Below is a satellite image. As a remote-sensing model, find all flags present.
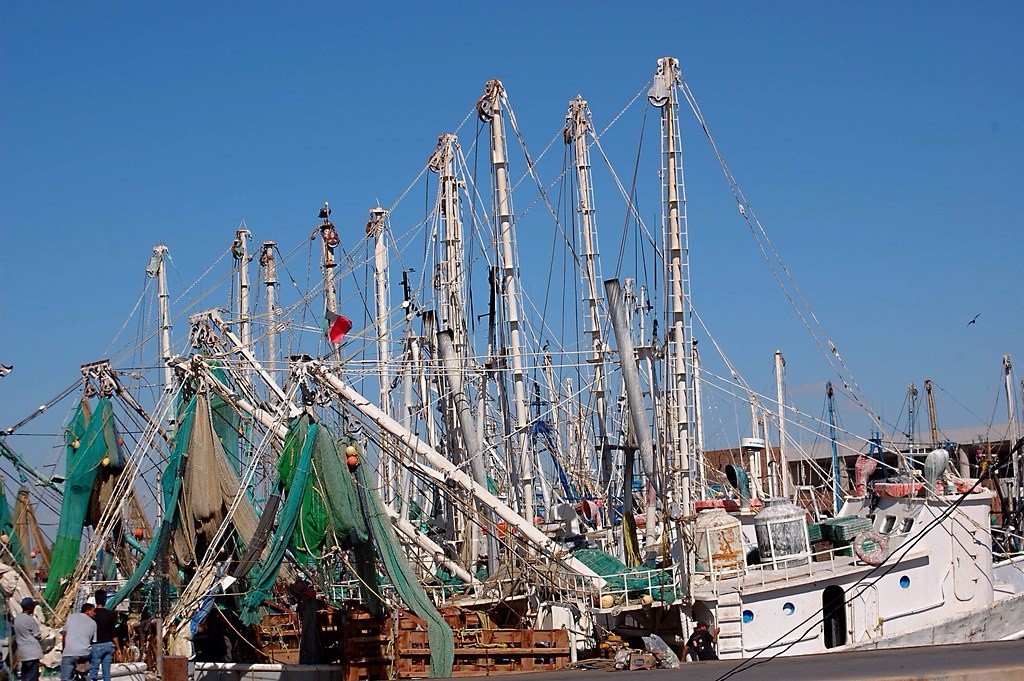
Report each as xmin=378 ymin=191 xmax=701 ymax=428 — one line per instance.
xmin=321 ymin=308 xmax=352 ymax=343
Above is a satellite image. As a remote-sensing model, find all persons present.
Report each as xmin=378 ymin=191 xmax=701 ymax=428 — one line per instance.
xmin=125 ymin=638 xmax=140 ymax=661
xmin=61 ymin=591 xmax=121 ymax=681
xmin=14 ymin=597 xmax=42 ymax=681
xmin=681 ymin=619 xmax=720 ymax=661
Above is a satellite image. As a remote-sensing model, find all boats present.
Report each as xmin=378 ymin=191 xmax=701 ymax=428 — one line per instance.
xmin=0 ymin=54 xmax=1024 ymax=660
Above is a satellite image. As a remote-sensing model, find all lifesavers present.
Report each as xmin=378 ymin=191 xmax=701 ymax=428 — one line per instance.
xmin=853 ymin=531 xmax=891 ymax=565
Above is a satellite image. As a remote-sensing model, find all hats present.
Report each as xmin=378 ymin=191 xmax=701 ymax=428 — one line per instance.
xmin=21 ymin=597 xmax=40 ymax=607
xmin=696 ymin=621 xmax=710 ymax=629
